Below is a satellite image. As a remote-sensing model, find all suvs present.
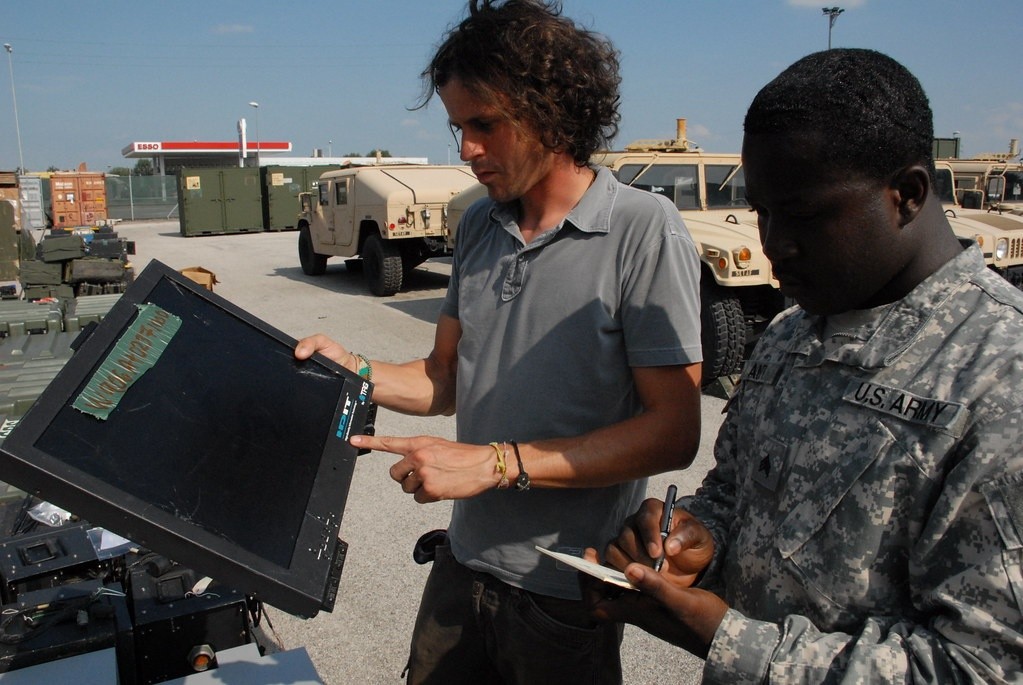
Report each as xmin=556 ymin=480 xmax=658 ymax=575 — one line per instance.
xmin=295 ymin=163 xmax=482 ymax=298
xmin=594 ymin=118 xmax=1023 ymax=393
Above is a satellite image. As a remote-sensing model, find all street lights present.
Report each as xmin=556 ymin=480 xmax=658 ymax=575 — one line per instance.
xmin=4 ymin=43 xmax=23 ymax=176
xmin=249 ymin=102 xmax=259 ymax=162
xmin=328 ymin=140 xmax=332 ymax=157
xmin=448 ymin=143 xmax=452 ymax=165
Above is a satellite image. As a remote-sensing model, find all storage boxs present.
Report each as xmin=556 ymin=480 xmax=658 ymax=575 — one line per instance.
xmin=259 ymin=164 xmax=341 ymax=233
xmin=51 ymin=174 xmax=108 ymax=228
xmin=17 ymin=176 xmax=46 ymax=230
xmin=20 ymin=224 xmax=136 ymax=300
xmin=176 ymin=266 xmax=222 ymax=292
xmin=176 ymin=168 xmax=266 ymax=237
xmin=0 ymin=172 xmax=21 ymax=232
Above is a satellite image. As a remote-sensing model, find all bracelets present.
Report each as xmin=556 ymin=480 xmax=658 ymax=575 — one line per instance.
xmin=350 ymin=350 xmax=373 ymax=381
xmin=490 ymin=438 xmax=530 ymax=492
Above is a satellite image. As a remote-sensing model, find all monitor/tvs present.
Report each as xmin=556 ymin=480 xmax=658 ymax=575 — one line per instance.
xmin=0 ymin=257 xmax=376 ymax=618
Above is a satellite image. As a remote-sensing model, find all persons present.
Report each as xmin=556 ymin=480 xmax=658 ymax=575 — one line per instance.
xmin=291 ymin=0 xmax=704 ymax=684
xmin=568 ymin=44 xmax=1023 ymax=684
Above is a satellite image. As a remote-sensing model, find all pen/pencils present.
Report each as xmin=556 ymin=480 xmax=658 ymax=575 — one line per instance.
xmin=654 ymin=483 xmax=678 ymax=572
